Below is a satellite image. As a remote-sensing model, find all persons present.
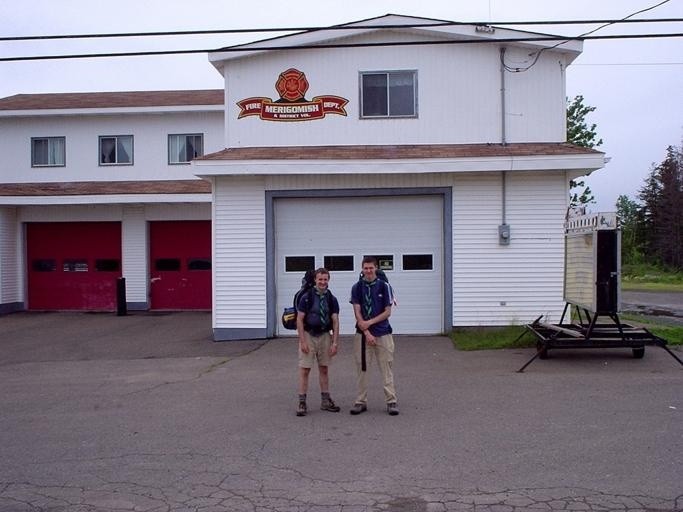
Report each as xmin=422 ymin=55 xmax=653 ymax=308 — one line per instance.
xmin=295 ymin=267 xmax=339 ymax=416
xmin=349 ymin=256 xmax=398 ymax=414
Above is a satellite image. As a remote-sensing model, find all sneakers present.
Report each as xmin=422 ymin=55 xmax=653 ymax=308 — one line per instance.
xmin=350 ymin=400 xmax=367 ymax=414
xmin=296 ymin=399 xmax=307 ymax=416
xmin=387 ymin=402 xmax=400 ymax=415
xmin=320 ymin=398 xmax=340 ymax=412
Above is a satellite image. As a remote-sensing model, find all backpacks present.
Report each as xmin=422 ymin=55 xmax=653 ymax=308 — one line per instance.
xmin=358 ymin=269 xmax=389 ymax=305
xmin=281 ymin=269 xmax=333 ymax=330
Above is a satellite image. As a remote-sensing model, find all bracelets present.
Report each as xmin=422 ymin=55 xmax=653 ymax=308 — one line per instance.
xmin=332 ymin=343 xmax=338 ymax=347
